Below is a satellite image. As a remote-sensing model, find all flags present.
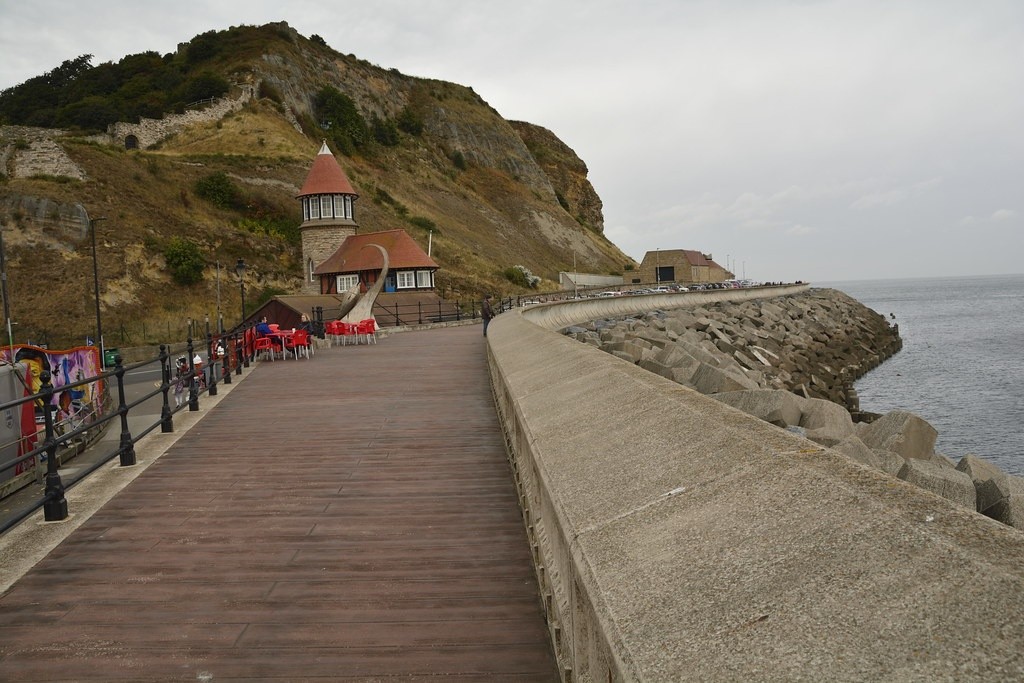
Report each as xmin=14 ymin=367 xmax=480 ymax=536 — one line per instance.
xmin=88 ymin=338 xmax=95 ymax=346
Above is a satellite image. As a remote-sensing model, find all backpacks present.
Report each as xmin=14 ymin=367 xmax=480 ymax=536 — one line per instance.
xmin=175 ymin=380 xmax=185 ymax=393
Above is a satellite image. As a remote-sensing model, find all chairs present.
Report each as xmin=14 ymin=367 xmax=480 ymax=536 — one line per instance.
xmin=324 ymin=319 xmax=377 ymax=346
xmin=252 ymin=324 xmax=314 ymax=362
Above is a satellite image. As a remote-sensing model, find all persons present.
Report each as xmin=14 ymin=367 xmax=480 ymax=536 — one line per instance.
xmin=292 ymin=313 xmax=312 ymax=355
xmin=255 ymin=316 xmax=294 ymax=355
xmin=172 ymin=373 xmax=184 ymax=408
xmin=482 ymin=294 xmax=495 ymax=337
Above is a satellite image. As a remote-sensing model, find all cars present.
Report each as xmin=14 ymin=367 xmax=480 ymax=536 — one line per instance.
xmin=600 ymin=279 xmax=759 ymax=300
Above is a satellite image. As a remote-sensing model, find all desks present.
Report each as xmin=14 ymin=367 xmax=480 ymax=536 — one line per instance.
xmin=266 ymin=333 xmax=291 ymax=362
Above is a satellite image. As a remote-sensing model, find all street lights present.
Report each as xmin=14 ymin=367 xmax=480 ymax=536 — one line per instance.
xmin=90 ymin=217 xmax=107 ymax=371
xmin=235 ymin=257 xmax=250 ymax=367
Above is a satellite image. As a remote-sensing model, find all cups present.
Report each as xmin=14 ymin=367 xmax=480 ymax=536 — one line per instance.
xmin=292 ymin=328 xmax=295 ymax=334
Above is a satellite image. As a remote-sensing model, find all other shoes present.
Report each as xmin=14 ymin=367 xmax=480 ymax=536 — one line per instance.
xmin=176 ymin=405 xmax=179 ymax=408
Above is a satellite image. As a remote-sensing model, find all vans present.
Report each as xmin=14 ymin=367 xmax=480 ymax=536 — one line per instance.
xmin=101 ymin=348 xmax=120 ymax=366
